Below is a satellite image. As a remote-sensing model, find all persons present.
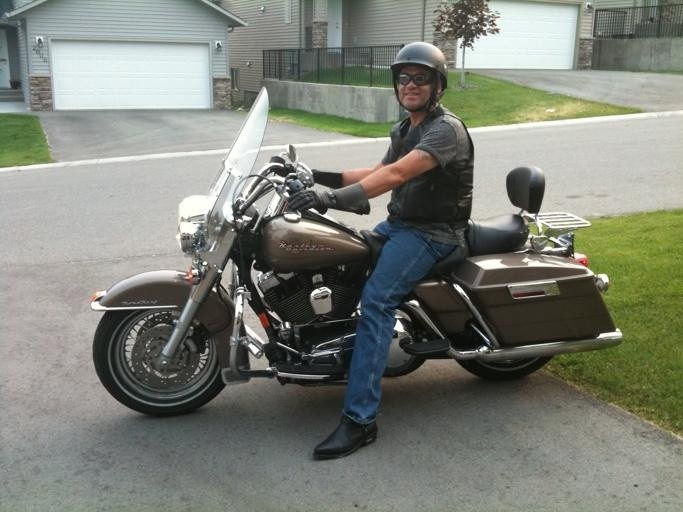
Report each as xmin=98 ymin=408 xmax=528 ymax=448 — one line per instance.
xmin=263 ymin=37 xmax=478 ymax=461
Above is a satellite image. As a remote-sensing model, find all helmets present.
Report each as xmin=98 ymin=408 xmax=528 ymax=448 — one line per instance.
xmin=390 ymin=41 xmax=448 ymax=89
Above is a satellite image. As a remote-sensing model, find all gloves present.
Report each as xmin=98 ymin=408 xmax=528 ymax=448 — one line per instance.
xmin=286 ymin=183 xmax=371 ymax=218
xmin=310 ymin=168 xmax=342 ymax=189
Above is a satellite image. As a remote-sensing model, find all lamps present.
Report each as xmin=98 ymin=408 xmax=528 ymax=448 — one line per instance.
xmin=36 ymin=36 xmax=43 ymax=47
xmin=215 ymin=41 xmax=222 ymax=52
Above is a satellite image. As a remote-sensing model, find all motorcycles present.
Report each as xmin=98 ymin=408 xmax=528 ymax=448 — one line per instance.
xmin=86 ymin=86 xmax=623 ymax=419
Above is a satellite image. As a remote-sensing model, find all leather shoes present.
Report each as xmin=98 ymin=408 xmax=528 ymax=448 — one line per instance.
xmin=312 ymin=417 xmax=379 ymax=460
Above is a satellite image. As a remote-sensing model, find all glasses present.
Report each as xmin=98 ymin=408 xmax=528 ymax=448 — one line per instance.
xmin=396 ymin=72 xmax=435 ymax=86
